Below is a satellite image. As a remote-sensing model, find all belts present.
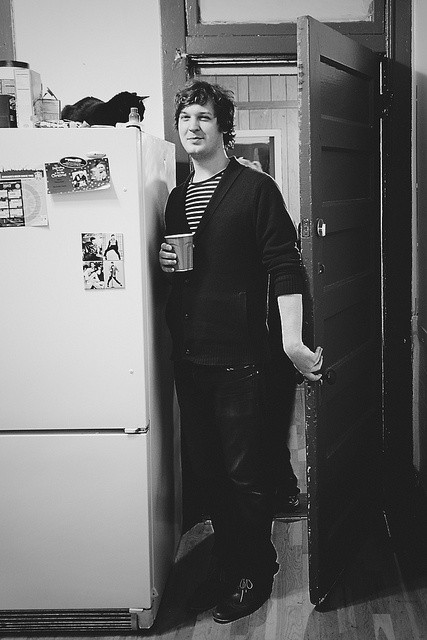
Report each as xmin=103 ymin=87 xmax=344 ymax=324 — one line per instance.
xmin=178 ymin=362 xmax=256 ymax=384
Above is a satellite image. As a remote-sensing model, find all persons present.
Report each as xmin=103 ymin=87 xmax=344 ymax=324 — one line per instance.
xmin=158 ymin=83 xmax=325 ymax=624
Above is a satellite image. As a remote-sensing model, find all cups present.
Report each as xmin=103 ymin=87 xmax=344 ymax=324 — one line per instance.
xmin=164 ymin=228 xmax=196 ymax=275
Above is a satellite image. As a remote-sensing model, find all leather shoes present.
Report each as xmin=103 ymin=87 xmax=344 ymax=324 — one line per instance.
xmin=189 ymin=571 xmax=227 ymax=614
xmin=213 ymin=574 xmax=272 ymax=625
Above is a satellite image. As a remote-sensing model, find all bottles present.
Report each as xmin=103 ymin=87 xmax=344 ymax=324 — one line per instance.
xmin=129 ymin=106 xmax=140 ymax=127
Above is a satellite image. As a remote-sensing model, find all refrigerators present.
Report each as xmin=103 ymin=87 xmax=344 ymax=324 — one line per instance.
xmin=1 ymin=126 xmax=187 ymax=635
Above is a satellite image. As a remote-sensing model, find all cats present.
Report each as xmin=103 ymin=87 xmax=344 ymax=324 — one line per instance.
xmin=60 ymin=90 xmax=150 ymax=128
